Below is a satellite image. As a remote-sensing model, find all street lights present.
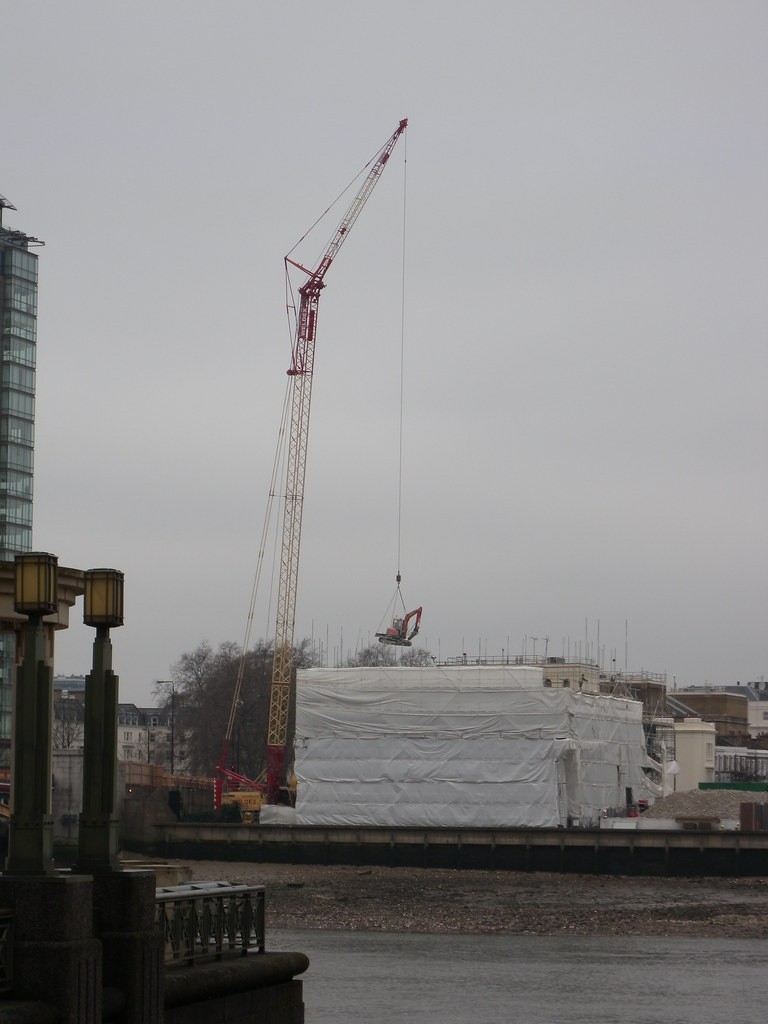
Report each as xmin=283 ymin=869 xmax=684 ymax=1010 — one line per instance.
xmin=4 ymin=550 xmax=61 ymax=876
xmin=73 ymin=565 xmax=127 ymax=874
xmin=62 ymin=698 xmax=79 ymax=748
xmin=142 ymin=726 xmax=150 ymax=765
xmin=156 ymin=680 xmax=175 ymax=776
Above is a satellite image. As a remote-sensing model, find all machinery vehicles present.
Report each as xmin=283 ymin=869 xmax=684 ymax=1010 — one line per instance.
xmin=205 ymin=111 xmax=413 ymax=826
xmin=375 ymin=605 xmax=424 ymax=647
